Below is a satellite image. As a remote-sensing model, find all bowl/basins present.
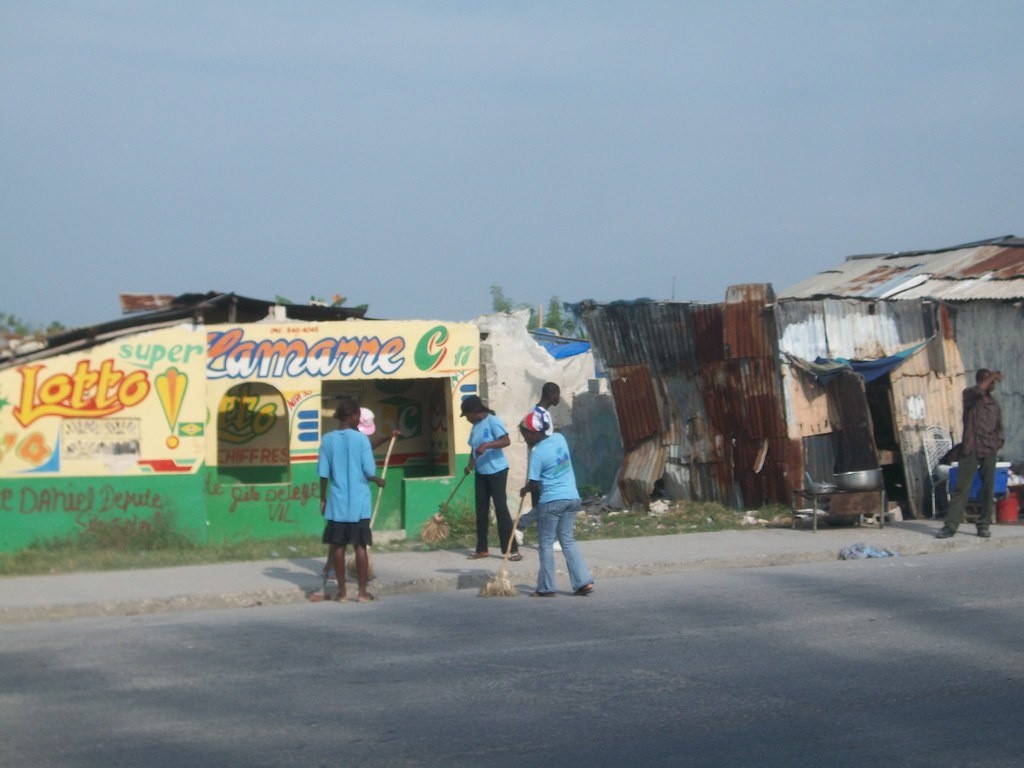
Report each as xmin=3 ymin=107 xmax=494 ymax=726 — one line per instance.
xmin=833 ymin=468 xmax=882 ymax=491
xmin=810 ymin=484 xmax=837 ymax=494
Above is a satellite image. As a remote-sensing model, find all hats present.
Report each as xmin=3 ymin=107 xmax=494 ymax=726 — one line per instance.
xmin=460 ymin=396 xmax=481 ymax=417
xmin=357 ymin=407 xmax=376 ymax=435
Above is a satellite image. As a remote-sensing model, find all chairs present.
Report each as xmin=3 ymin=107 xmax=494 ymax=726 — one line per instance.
xmin=920 ymin=426 xmax=998 ymax=524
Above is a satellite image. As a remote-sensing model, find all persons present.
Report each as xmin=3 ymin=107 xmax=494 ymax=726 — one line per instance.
xmin=935 ymin=368 xmax=1006 ymax=538
xmin=519 ymin=405 xmax=596 ymax=596
xmin=459 ymin=395 xmax=523 ymax=561
xmin=513 ymin=381 xmax=562 ymax=552
xmin=316 ymin=401 xmax=386 ymax=602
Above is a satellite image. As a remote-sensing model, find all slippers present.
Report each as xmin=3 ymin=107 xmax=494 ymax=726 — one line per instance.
xmin=530 ymin=591 xmax=556 ymax=597
xmin=574 ymin=588 xmax=593 ymax=595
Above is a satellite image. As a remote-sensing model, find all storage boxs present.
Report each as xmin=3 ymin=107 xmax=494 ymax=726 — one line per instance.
xmin=947 ymin=461 xmax=1012 ymax=503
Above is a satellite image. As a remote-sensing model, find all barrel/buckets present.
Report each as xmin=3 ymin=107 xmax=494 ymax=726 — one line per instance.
xmin=996 ymin=492 xmax=1019 ymax=523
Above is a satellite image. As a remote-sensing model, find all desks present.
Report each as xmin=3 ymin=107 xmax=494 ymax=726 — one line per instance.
xmin=790 ymin=488 xmax=885 ymax=534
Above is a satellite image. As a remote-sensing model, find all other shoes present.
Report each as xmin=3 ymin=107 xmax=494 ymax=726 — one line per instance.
xmin=978 ymin=530 xmax=991 ymax=537
xmin=937 ymin=526 xmax=953 ymax=538
xmin=337 ymin=592 xmax=347 ymax=602
xmin=361 ymin=593 xmax=374 ymax=602
xmin=509 ymin=552 xmax=521 ymax=561
xmin=467 ymin=552 xmax=488 ymax=559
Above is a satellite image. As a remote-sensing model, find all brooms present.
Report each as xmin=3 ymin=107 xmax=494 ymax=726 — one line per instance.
xmin=420 ymin=454 xmax=480 ymax=544
xmin=477 ymin=479 xmax=530 ymax=598
xmin=309 ymin=543 xmax=334 ymax=603
xmin=345 ymin=428 xmax=402 ymax=582
